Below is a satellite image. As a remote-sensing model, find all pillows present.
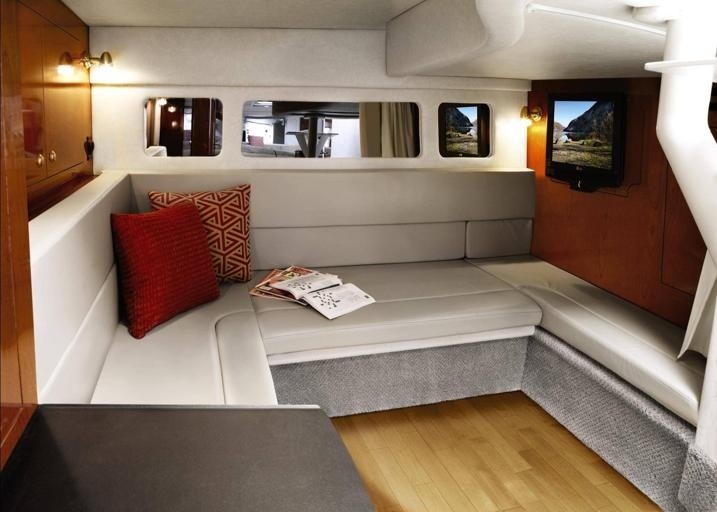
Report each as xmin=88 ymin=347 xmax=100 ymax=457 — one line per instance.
xmin=110 ymin=201 xmax=220 ymax=337
xmin=148 ymin=185 xmax=251 ymax=284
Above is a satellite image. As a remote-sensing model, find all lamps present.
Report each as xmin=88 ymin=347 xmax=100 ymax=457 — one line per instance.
xmin=56 ymin=50 xmax=82 ymax=78
xmin=520 ymin=101 xmax=541 ymax=124
xmin=82 ymin=50 xmax=114 ymax=69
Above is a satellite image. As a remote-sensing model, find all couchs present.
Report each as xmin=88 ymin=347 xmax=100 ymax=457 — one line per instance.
xmin=28 ymin=163 xmax=717 ymax=509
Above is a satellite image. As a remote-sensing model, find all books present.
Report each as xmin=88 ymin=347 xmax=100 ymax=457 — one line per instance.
xmin=249 ymin=264 xmax=376 ymax=321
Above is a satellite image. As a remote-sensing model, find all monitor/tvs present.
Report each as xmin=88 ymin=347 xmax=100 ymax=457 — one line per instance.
xmin=543 ymin=92 xmax=628 ymax=192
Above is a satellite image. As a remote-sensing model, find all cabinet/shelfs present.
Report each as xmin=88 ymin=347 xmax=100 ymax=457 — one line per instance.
xmin=15 ymin=1 xmax=89 ymax=196
xmin=0 ymin=0 xmax=37 ymax=484
xmin=661 ymin=148 xmax=707 ymax=296
